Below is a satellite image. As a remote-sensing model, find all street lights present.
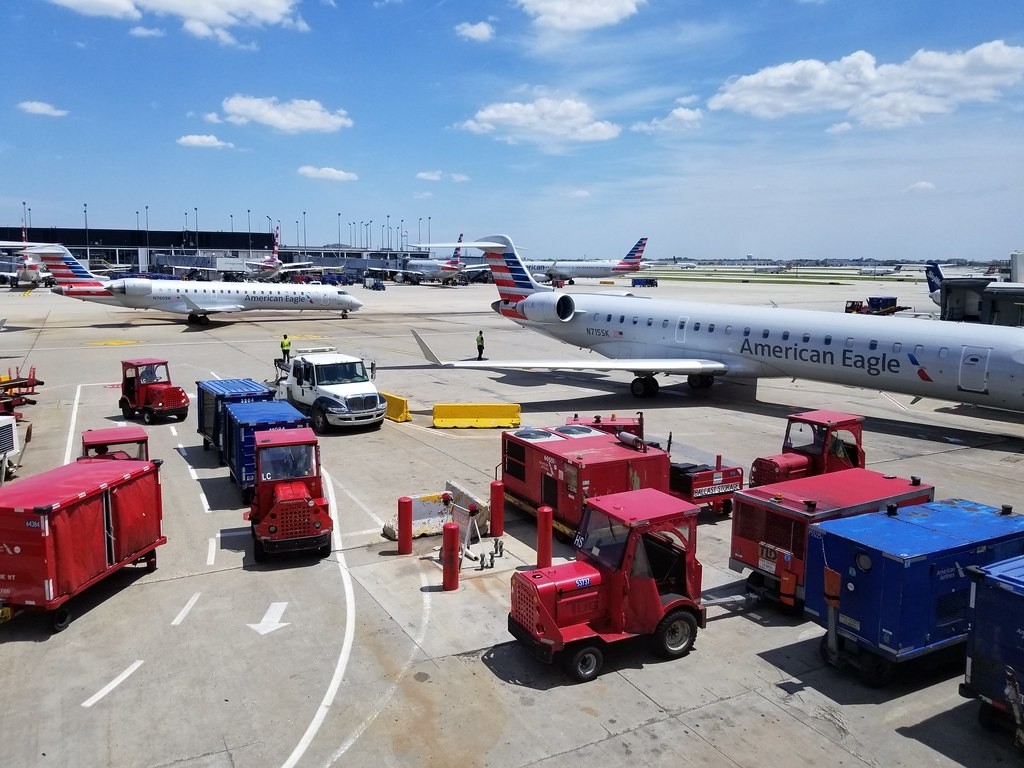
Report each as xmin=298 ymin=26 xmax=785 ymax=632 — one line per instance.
xmin=22 ymin=202 xmax=32 ymax=242
xmin=83 ymin=203 xmax=89 ymax=260
xmin=184 ymin=212 xmax=188 ymax=240
xmin=195 ymin=208 xmax=198 ymax=249
xmin=135 ymin=211 xmax=139 ymax=231
xmin=230 ymin=214 xmax=233 ymax=232
xmin=266 ymin=215 xmax=272 ymax=233
xmin=247 ymin=209 xmax=251 ymax=249
xmin=337 ymin=213 xmax=341 ymax=251
xmin=348 ymin=214 xmax=431 ymax=249
xmin=145 ymin=206 xmax=148 ymax=248
xmin=295 ymin=211 xmax=306 ymax=256
xmin=277 ymin=219 xmax=281 ymax=246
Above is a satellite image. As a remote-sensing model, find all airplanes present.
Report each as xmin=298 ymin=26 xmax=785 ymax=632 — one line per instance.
xmin=244 ymin=257 xmax=318 ymax=284
xmin=17 ymin=244 xmax=365 ymax=326
xmin=522 ymin=238 xmax=660 ymax=285
xmin=0 ymin=257 xmax=53 ymax=288
xmin=753 ymin=262 xmax=793 ymax=274
xmin=370 ymin=234 xmax=492 ymax=286
xmin=857 ymin=264 xmax=901 ymax=276
xmin=403 ymin=235 xmax=1024 ymax=412
xmin=666 ymin=256 xmax=696 ymax=270
xmin=892 ymin=261 xmax=1024 ymax=307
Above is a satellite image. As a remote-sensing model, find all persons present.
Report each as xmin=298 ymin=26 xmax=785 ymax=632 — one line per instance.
xmin=94 ymin=446 xmax=117 ymax=460
xmin=476 ymin=330 xmax=485 ymax=360
xmin=281 ymin=334 xmax=291 ymax=364
xmin=141 ymin=367 xmax=155 ymax=383
xmin=282 ymin=445 xmax=310 ymax=476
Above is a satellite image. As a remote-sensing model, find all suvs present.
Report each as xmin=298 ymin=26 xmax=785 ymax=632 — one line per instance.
xmin=287 ymin=353 xmax=388 ymax=433
